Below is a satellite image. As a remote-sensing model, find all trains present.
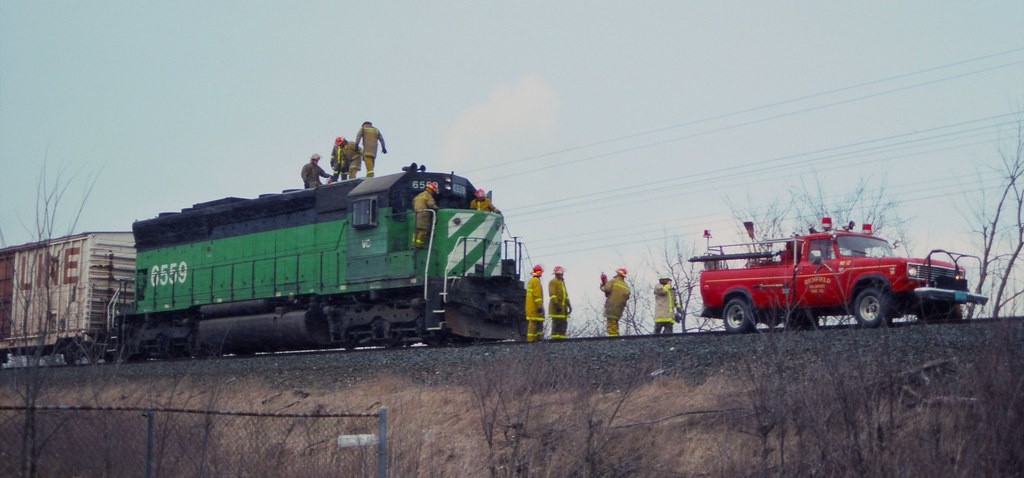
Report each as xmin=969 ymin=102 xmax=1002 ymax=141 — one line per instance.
xmin=0 ymin=162 xmax=526 ymax=364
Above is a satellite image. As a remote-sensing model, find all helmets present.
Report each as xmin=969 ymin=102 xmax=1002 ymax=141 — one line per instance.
xmin=310 ymin=154 xmax=320 ymax=160
xmin=531 ymin=264 xmax=544 ymax=273
xmin=552 ymin=266 xmax=564 ymax=273
xmin=428 ymin=183 xmax=436 ymax=190
xmin=616 ymin=269 xmax=628 ymax=277
xmin=335 ymin=137 xmax=345 ymax=145
xmin=475 ymin=189 xmax=485 ymax=197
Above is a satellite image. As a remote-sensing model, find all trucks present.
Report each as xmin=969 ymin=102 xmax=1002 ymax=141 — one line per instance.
xmin=688 ymin=217 xmax=989 ymax=327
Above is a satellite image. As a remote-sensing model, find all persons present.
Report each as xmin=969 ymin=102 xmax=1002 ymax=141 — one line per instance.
xmin=336 ymin=137 xmax=364 ymax=180
xmin=469 ymin=188 xmax=503 ymax=215
xmin=525 ymin=264 xmax=546 ymax=342
xmin=301 ymin=153 xmax=333 ymax=190
xmin=330 ymin=145 xmax=348 ymax=183
xmin=599 ymin=268 xmax=630 ymax=337
xmin=652 ymin=271 xmax=685 ymax=334
xmin=548 ymin=265 xmax=572 ymax=339
xmin=411 ymin=182 xmax=437 ymax=249
xmin=356 ymin=121 xmax=388 ymax=178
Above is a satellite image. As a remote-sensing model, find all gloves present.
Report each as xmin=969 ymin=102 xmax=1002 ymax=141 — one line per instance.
xmin=382 ymin=149 xmax=387 ymax=154
xmin=356 ymin=145 xmax=358 ymax=151
xmin=677 ymin=308 xmax=687 ymax=316
xmin=675 ymin=314 xmax=682 ymax=323
xmin=538 ymin=308 xmax=543 ymax=313
xmin=555 ymin=304 xmax=561 ymax=312
